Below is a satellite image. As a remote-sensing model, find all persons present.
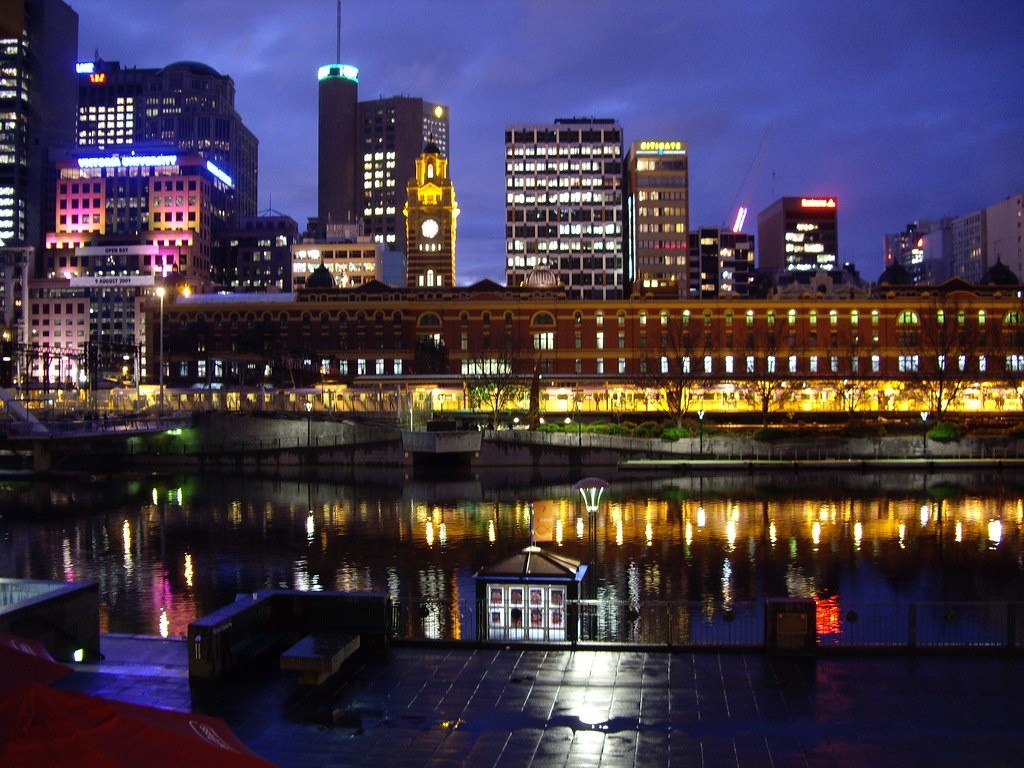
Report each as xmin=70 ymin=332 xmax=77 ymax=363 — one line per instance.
xmin=83 ymin=410 xmax=116 ymax=431
xmin=128 ymin=411 xmax=137 ymax=419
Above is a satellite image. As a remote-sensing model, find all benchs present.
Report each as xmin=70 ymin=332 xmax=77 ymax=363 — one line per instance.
xmin=222 ymin=606 xmax=293 ymax=682
xmin=293 ymin=603 xmax=405 ymax=654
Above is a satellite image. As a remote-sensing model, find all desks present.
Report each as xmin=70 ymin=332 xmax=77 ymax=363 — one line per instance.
xmin=280 ymin=632 xmax=362 ymax=685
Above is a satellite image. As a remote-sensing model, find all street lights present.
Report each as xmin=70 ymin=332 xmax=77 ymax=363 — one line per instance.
xmin=920 ymin=411 xmax=929 ymax=459
xmin=575 ymin=477 xmax=607 ymax=642
xmin=697 ymin=409 xmax=705 ymax=460
xmin=154 ymin=284 xmax=191 ymax=417
